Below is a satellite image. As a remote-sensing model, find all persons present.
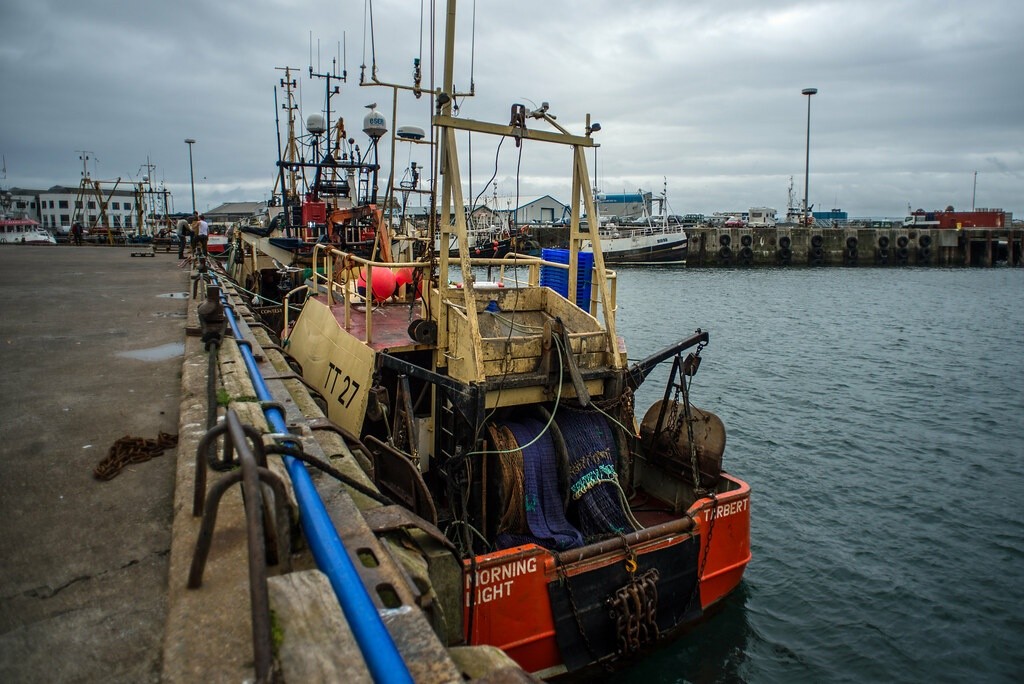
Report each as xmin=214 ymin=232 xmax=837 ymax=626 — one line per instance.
xmin=71 ymin=221 xmax=84 ymax=246
xmin=176 ymin=215 xmax=195 ymax=259
xmin=194 ymin=215 xmax=209 ymax=256
xmin=189 ymin=212 xmax=204 ymax=254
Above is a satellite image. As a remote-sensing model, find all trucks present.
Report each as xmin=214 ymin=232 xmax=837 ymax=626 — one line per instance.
xmin=901 ymin=215 xmax=940 ymax=229
xmin=748 ymin=206 xmax=777 ymax=229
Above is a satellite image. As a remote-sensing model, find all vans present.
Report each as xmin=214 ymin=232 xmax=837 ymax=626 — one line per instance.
xmin=741 ymin=216 xmax=749 ymax=225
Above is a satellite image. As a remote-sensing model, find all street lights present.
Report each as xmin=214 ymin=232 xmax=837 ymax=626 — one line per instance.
xmin=184 ymin=139 xmax=196 ymax=212
xmin=415 ymin=165 xmax=423 ymax=206
xmin=802 ymin=88 xmax=818 ymax=227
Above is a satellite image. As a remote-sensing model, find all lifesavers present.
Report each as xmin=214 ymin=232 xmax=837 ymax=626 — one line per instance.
xmin=740 ymin=234 xmax=754 ymax=268
xmin=846 ymin=237 xmax=860 ymax=268
xmin=778 ymin=236 xmax=793 ymax=265
xmin=919 ymin=234 xmax=933 ymax=267
xmin=897 ymin=236 xmax=910 ymax=268
xmin=811 ymin=234 xmax=825 ymax=267
xmin=718 ymin=234 xmax=733 ymax=266
xmin=878 ymin=235 xmax=891 ymax=266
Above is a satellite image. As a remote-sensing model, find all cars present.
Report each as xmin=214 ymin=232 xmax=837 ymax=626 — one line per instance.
xmin=553 ymin=215 xmax=684 ymax=228
xmin=724 ymin=217 xmax=744 ymax=228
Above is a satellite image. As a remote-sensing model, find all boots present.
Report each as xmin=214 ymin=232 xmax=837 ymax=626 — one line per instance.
xmin=179 ymin=252 xmax=188 ymax=259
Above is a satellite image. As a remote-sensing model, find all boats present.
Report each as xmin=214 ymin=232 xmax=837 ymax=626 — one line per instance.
xmin=581 ymin=178 xmax=688 ymax=261
xmin=219 ymin=0 xmax=754 ymax=682
xmin=0 ymin=187 xmax=57 ymax=245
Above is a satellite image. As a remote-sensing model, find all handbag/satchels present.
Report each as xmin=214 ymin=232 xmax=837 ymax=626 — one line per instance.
xmin=190 ymin=229 xmax=194 ymax=237
xmin=182 ymin=225 xmax=191 ymax=236
